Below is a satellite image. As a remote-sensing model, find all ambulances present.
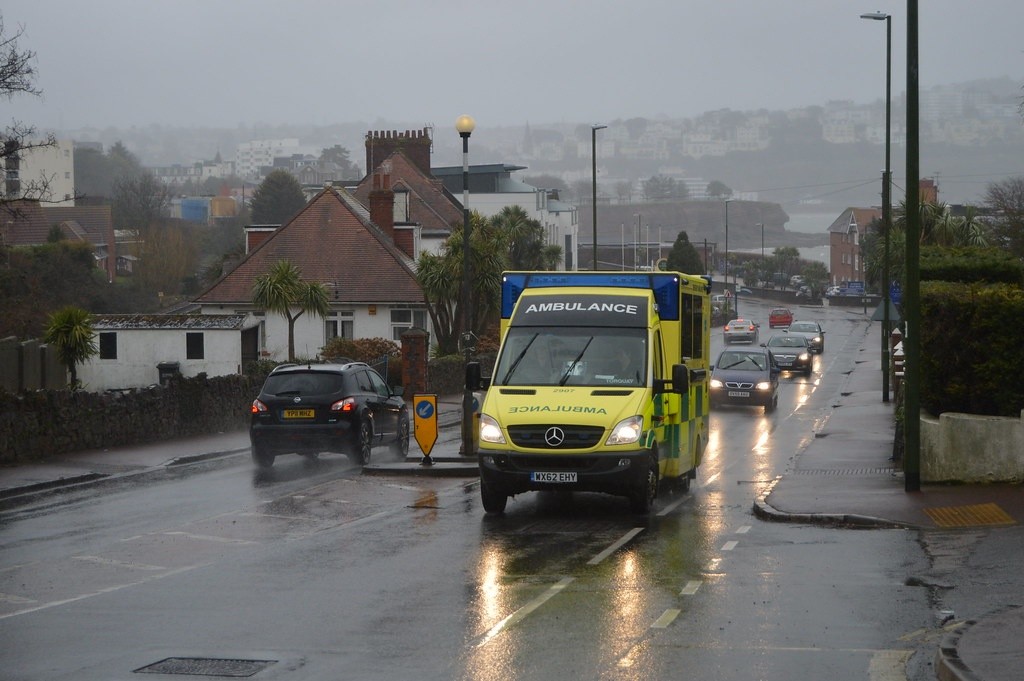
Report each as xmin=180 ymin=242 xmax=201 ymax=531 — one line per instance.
xmin=467 ymin=270 xmax=711 ymax=513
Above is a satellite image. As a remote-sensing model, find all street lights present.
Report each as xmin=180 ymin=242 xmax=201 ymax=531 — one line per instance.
xmin=725 ymin=198 xmax=732 ymax=326
xmin=632 ymin=212 xmax=641 ymax=269
xmin=592 ymin=125 xmax=609 ymax=271
xmin=861 ymin=13 xmax=892 ymax=403
xmin=756 ymin=223 xmax=765 ymax=293
xmin=457 ymin=113 xmax=474 ymax=454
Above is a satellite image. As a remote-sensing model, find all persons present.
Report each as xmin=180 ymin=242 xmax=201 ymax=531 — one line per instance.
xmin=611 ymin=348 xmax=640 ymax=380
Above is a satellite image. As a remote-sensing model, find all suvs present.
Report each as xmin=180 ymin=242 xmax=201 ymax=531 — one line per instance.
xmin=249 ymin=362 xmax=409 ymax=469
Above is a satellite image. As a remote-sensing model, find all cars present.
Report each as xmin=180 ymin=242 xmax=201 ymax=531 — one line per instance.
xmin=790 ymin=275 xmax=804 ymax=289
xmin=769 ymin=308 xmax=794 ymax=327
xmin=782 ymin=322 xmax=825 ymax=354
xmin=712 ymin=295 xmax=734 ymax=307
xmin=796 ymin=286 xmax=811 ymax=297
xmin=757 ymin=278 xmax=775 ymax=289
xmin=710 ymin=346 xmax=780 ymax=412
xmin=761 ymin=333 xmax=815 ymax=376
xmin=826 ymin=285 xmax=840 ymax=298
xmin=724 ymin=318 xmax=760 ymax=345
